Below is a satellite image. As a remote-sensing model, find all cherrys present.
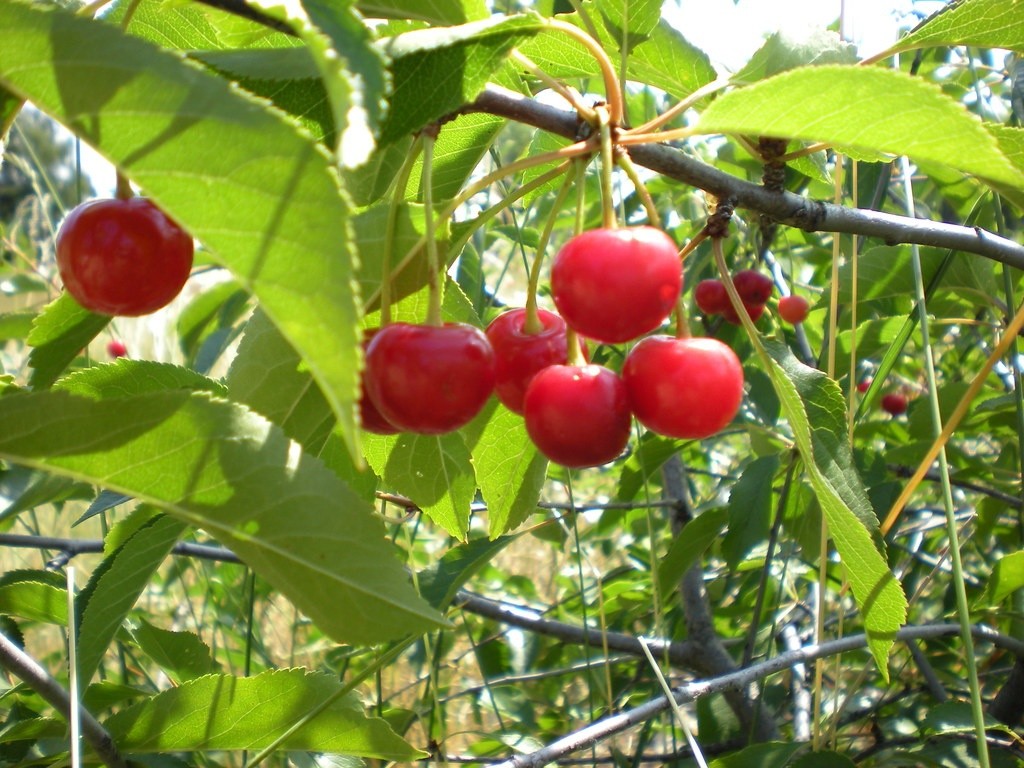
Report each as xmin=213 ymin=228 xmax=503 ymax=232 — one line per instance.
xmin=622 ymin=156 xmax=741 ymax=438
xmin=779 ymin=230 xmax=809 ymax=324
xmin=729 ymin=299 xmax=765 ymax=324
xmin=56 ymin=172 xmax=192 ymax=315
xmin=734 ymin=233 xmax=774 ymax=304
xmin=857 ymin=380 xmax=870 ymax=391
xmin=487 ymin=154 xmax=590 ymax=413
xmin=523 ymin=164 xmax=632 ymax=467
xmin=367 ymin=137 xmax=492 ymax=431
xmin=360 ymin=138 xmax=420 ymax=435
xmin=552 ymin=101 xmax=680 ymax=341
xmin=883 ymin=395 xmax=906 ymax=414
xmin=695 ymin=276 xmax=728 ymax=312
